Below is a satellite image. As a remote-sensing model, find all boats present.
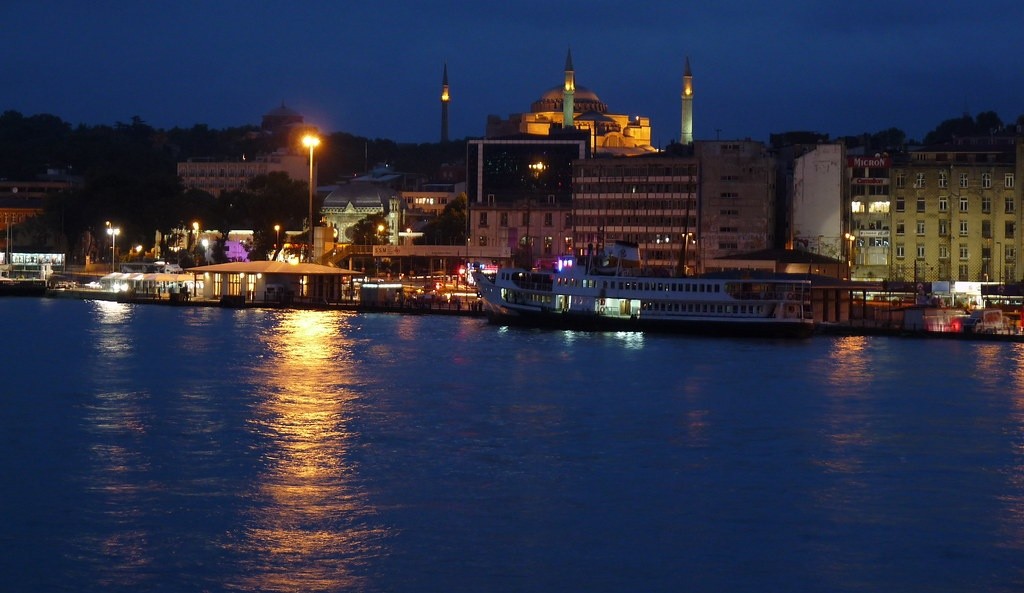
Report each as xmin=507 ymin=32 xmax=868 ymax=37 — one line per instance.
xmin=471 ymin=240 xmax=816 ymax=341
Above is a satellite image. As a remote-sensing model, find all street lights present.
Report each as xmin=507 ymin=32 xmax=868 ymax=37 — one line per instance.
xmin=984 ymin=273 xmax=988 ymax=299
xmin=996 ymin=241 xmax=1001 ymax=304
xmin=300 ymin=133 xmax=321 ymax=263
xmin=817 ymin=234 xmax=826 ymax=255
xmin=843 ymin=232 xmax=855 ymax=281
xmin=274 ymin=224 xmax=280 ymax=248
xmin=106 ymin=228 xmax=120 ymax=274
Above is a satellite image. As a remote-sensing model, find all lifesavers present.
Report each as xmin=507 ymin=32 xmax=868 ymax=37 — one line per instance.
xmin=789 ymin=306 xmax=794 ymax=313
xmin=600 ymin=307 xmax=605 ymax=313
xmin=787 ymin=293 xmax=796 ymax=300
